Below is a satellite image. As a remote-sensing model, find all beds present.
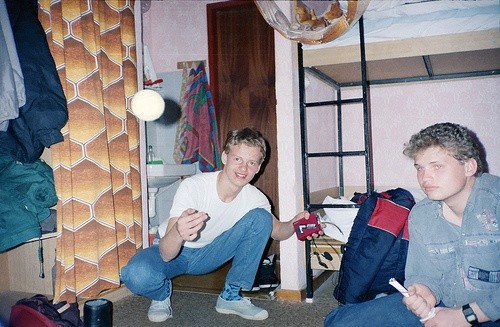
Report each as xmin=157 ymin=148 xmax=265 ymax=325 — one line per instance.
xmin=293 ymin=0 xmax=500 ymax=304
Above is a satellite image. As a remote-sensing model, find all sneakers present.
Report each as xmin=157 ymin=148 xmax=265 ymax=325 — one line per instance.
xmin=215 ymin=295 xmax=269 ymax=321
xmin=147 ymin=280 xmax=172 ymax=322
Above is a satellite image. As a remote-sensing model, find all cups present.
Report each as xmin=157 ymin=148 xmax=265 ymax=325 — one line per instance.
xmin=84 ymin=299 xmax=113 ymax=327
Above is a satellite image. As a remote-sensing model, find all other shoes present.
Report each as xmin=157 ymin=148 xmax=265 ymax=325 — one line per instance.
xmin=258 ymin=254 xmax=278 ymax=288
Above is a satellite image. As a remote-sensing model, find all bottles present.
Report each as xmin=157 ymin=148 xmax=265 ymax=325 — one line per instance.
xmin=147 ymin=145 xmax=154 ymax=162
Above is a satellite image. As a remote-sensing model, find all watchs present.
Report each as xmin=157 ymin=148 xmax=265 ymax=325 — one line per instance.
xmin=462 ymin=303 xmax=480 ymax=327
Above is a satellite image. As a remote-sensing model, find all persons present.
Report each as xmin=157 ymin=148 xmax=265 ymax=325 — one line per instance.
xmin=324 ymin=122 xmax=500 ymax=327
xmin=121 ymin=127 xmax=326 ymax=323
